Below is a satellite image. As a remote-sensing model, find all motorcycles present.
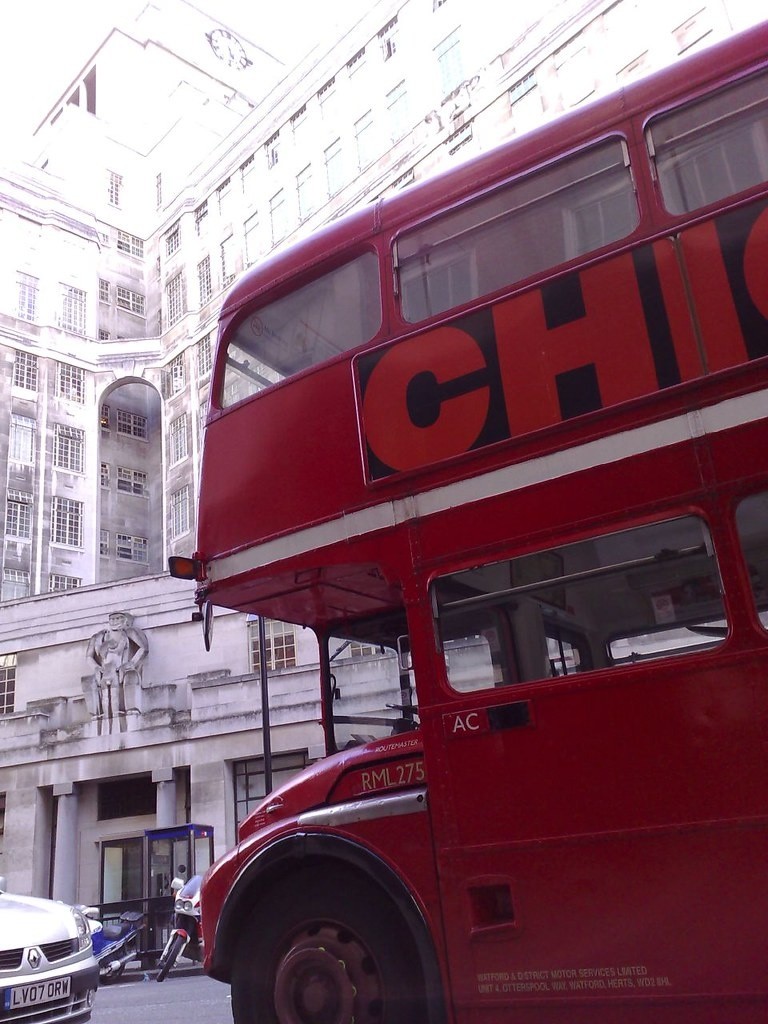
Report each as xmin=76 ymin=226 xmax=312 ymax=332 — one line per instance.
xmin=73 ymin=904 xmax=146 ymax=984
xmin=156 ymin=875 xmax=203 ymax=983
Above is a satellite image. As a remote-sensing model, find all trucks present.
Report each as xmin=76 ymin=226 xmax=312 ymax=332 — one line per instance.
xmin=170 ymin=16 xmax=766 ymax=1023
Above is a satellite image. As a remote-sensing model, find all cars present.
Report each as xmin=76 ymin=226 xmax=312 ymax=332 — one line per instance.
xmin=0 ymin=875 xmax=99 ymax=1024
